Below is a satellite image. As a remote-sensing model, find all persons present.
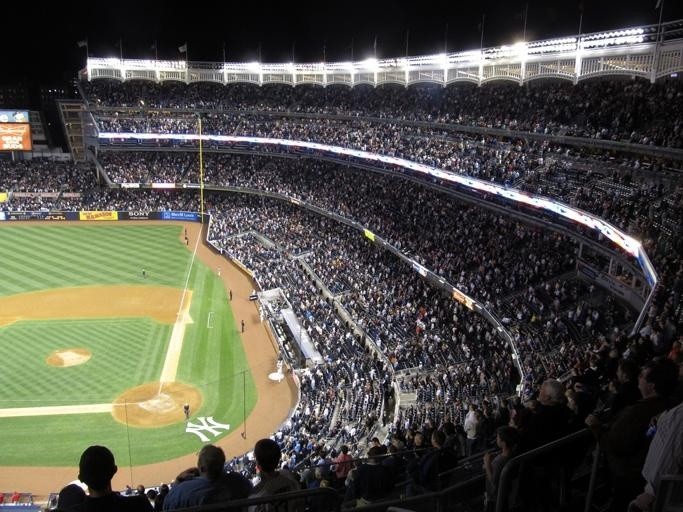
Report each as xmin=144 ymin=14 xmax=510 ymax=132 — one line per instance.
xmin=202 ymin=220 xmax=681 ymax=512
xmin=183 ymin=402 xmax=190 ymax=418
xmin=0 ymin=76 xmax=682 ymax=219
xmin=0 ymin=491 xmax=22 ymax=506
xmin=45 ymin=444 xmax=203 ymax=512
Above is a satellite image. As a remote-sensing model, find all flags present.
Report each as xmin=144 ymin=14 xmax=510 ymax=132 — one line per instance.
xmin=177 ymin=45 xmax=186 ymax=52
xmin=76 ymin=40 xmax=87 ymax=47
